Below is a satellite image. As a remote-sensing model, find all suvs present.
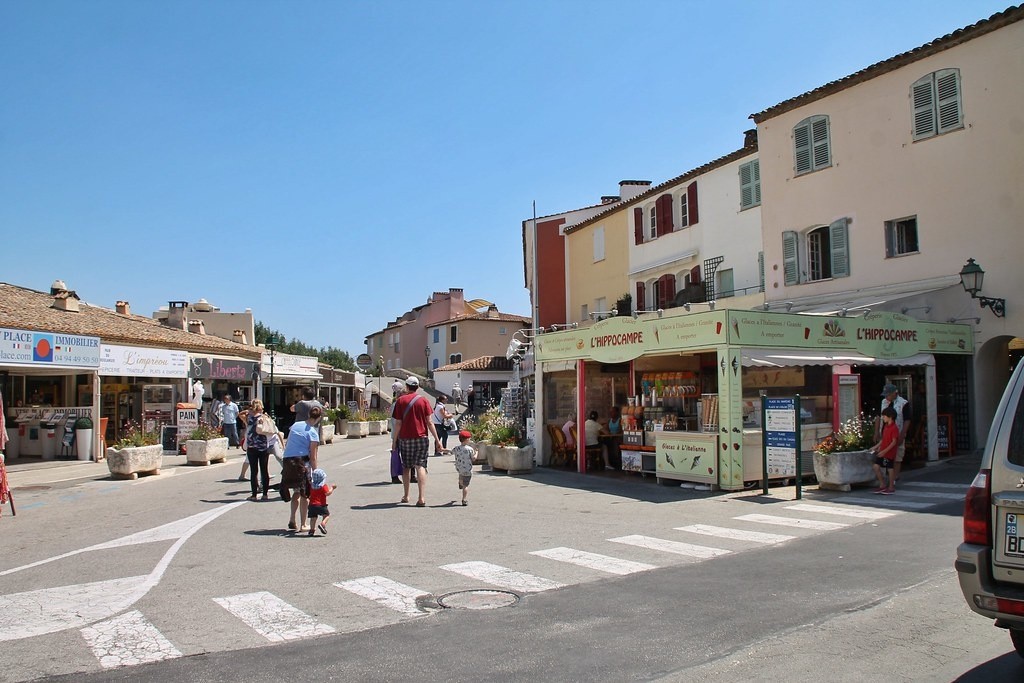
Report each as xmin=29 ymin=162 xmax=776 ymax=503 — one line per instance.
xmin=954 ymin=355 xmax=1024 ymax=663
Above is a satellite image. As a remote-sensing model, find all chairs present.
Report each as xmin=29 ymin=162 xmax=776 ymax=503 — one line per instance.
xmin=547 ymin=423 xmax=604 ymax=469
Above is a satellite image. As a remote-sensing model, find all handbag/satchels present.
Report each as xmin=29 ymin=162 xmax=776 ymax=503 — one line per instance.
xmin=390 ymin=447 xmax=404 ymax=477
xmin=256 ymin=413 xmax=277 ymax=435
xmin=433 ymin=404 xmax=452 ymax=430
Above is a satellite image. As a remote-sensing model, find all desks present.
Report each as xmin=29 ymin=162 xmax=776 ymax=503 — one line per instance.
xmin=599 ymin=434 xmax=624 ymax=437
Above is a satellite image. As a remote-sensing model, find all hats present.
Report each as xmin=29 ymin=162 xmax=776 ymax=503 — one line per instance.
xmin=881 ymin=383 xmax=896 ymax=396
xmin=311 ymin=468 xmax=327 ymax=489
xmin=459 ymin=431 xmax=472 ymax=438
xmin=405 ymin=376 xmax=419 ymax=386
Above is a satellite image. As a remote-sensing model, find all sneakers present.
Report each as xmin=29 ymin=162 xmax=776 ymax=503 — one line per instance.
xmin=871 ymin=486 xmax=887 ymax=494
xmin=459 ymin=483 xmax=464 ymax=489
xmin=880 ymin=486 xmax=895 ymax=495
xmin=318 ymin=523 xmax=327 ymax=534
xmin=462 ymin=500 xmax=468 ymax=506
xmin=308 ymin=529 xmax=315 ymax=535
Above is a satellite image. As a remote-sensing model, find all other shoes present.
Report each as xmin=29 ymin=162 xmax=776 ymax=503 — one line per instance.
xmin=260 ymin=497 xmax=268 ymax=501
xmin=247 ymin=496 xmax=256 ymax=501
xmin=239 ymin=476 xmax=249 ymax=480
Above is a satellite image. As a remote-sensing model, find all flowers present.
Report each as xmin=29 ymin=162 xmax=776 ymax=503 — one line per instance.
xmin=112 ymin=411 xmax=167 ymax=450
xmin=181 ymin=409 xmax=225 ymax=441
xmin=810 ymin=400 xmax=878 ymax=458
xmin=459 ymin=404 xmax=532 ymax=448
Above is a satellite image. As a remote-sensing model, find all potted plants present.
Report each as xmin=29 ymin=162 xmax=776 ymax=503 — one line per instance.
xmin=74 ymin=417 xmax=93 ymax=460
xmin=318 ymin=407 xmax=337 ymax=445
xmin=345 ymin=406 xmax=393 ymax=439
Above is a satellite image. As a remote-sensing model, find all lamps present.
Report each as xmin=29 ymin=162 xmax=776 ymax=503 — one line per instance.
xmin=958 ymin=255 xmax=1006 ymax=318
xmin=505 ymin=299 xmax=716 ymax=366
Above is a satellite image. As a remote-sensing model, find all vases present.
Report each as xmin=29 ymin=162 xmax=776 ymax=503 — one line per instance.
xmin=106 ymin=444 xmax=163 ymax=481
xmin=486 ymin=444 xmax=534 ymax=476
xmin=469 ymin=438 xmax=490 ymax=465
xmin=813 ymin=450 xmax=887 ymax=492
xmin=186 ymin=437 xmax=230 ymax=466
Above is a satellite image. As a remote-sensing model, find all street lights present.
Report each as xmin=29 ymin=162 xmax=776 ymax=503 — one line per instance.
xmin=377 ymin=358 xmax=385 ymax=411
xmin=424 ymin=345 xmax=431 ymax=379
xmin=265 ymin=334 xmax=281 ymax=424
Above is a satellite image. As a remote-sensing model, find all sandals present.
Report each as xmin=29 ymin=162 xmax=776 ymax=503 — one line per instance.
xmin=401 ymin=498 xmax=408 ymax=503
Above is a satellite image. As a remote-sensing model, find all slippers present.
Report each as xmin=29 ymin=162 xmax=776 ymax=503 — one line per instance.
xmin=300 ymin=525 xmax=311 ymax=532
xmin=416 ymin=501 xmax=425 ymax=506
xmin=289 ymin=521 xmax=299 ymax=529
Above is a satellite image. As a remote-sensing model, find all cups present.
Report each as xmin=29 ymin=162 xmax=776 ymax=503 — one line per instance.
xmin=641 ymin=393 xmax=646 ymax=406
xmin=651 ymin=388 xmax=657 ymax=406
xmin=635 ymin=394 xmax=640 ymax=406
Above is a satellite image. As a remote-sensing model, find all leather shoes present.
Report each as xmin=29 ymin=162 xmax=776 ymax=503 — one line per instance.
xmin=605 ymin=465 xmax=615 ymax=471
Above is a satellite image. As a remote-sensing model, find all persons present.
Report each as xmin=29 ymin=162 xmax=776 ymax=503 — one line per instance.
xmin=390 ymin=389 xmax=420 ymax=483
xmin=432 ymin=394 xmax=453 ymax=456
xmin=307 ymin=468 xmax=337 ymax=535
xmin=482 ymin=386 xmax=491 ymax=404
xmin=209 ymin=392 xmax=284 ymax=480
xmin=238 ymin=399 xmax=276 ymax=502
xmin=282 ymin=407 xmax=323 ymax=531
xmin=562 ymin=412 xmax=577 ymax=469
xmin=463 ymin=385 xmax=477 ymax=412
xmin=289 ymin=387 xmax=323 ymax=428
xmin=451 ymin=383 xmax=463 ymax=415
xmin=599 ymin=407 xmax=622 ymax=436
xmin=391 ymin=379 xmax=403 ymax=399
xmin=868 ymin=407 xmax=901 ymax=494
xmin=913 ymin=383 xmax=928 ymax=435
xmin=584 ymin=410 xmax=616 ymax=470
xmin=391 ymin=376 xmax=444 ymax=507
xmin=379 ymin=355 xmax=386 ymax=377
xmin=193 ymin=381 xmax=205 ymax=408
xmin=440 ymin=430 xmax=479 ymax=505
xmin=871 ymin=383 xmax=911 ymax=487
